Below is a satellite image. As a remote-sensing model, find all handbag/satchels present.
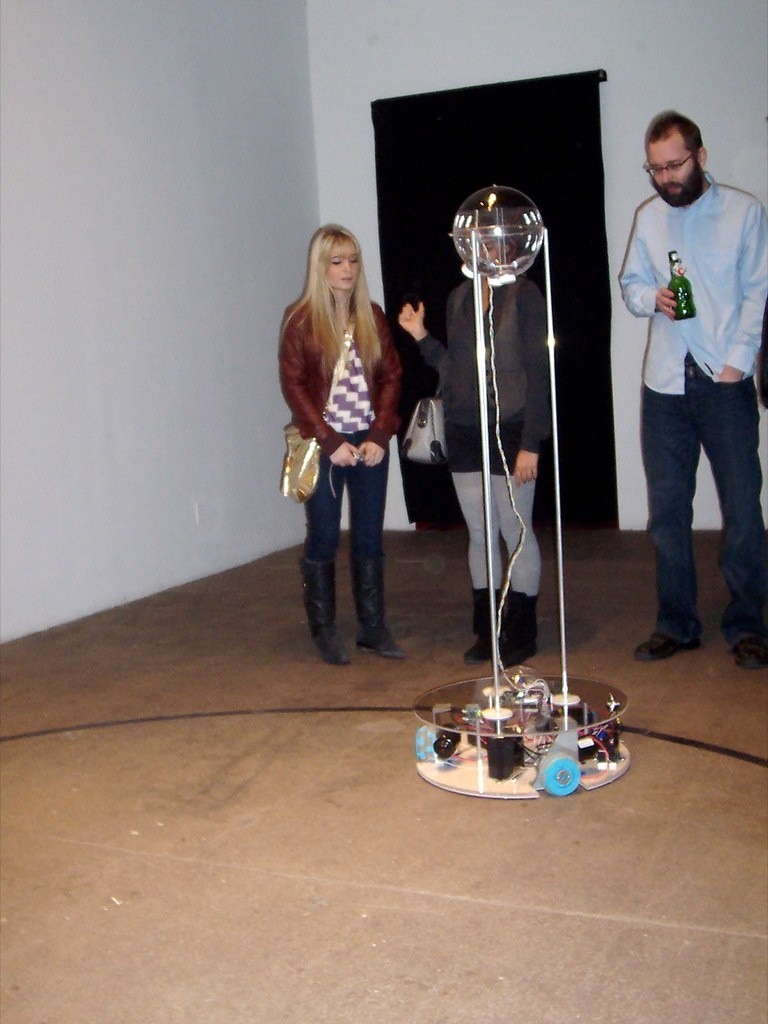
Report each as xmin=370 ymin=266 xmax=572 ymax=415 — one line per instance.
xmin=279 ymin=421 xmax=322 ymax=502
xmin=400 ymin=350 xmax=448 ymax=466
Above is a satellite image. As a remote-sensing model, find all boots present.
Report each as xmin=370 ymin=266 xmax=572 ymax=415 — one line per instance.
xmin=464 ymin=587 xmax=502 ymax=665
xmin=300 ymin=556 xmax=351 ymax=665
xmin=495 ymin=589 xmax=538 ymax=668
xmin=350 ymin=551 xmax=404 ymax=658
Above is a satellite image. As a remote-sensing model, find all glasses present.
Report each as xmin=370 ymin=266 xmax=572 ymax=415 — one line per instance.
xmin=646 ymin=150 xmax=696 ymax=177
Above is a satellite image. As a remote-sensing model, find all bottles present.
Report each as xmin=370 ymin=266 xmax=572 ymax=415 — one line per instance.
xmin=668 ymin=249 xmax=697 ymax=320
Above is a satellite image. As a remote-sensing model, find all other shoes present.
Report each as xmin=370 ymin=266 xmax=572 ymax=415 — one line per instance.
xmin=634 ymin=632 xmax=700 ymax=661
xmin=733 ymin=632 xmax=768 ymax=668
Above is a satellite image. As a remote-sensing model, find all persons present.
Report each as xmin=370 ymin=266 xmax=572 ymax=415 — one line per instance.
xmin=278 ymin=223 xmax=406 ymax=665
xmin=620 ymin=112 xmax=768 ymax=668
xmin=399 ymin=217 xmax=548 ymax=670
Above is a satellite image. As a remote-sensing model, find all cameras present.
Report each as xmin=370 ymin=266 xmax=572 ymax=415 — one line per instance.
xmin=352 ymin=452 xmax=366 ymax=462
